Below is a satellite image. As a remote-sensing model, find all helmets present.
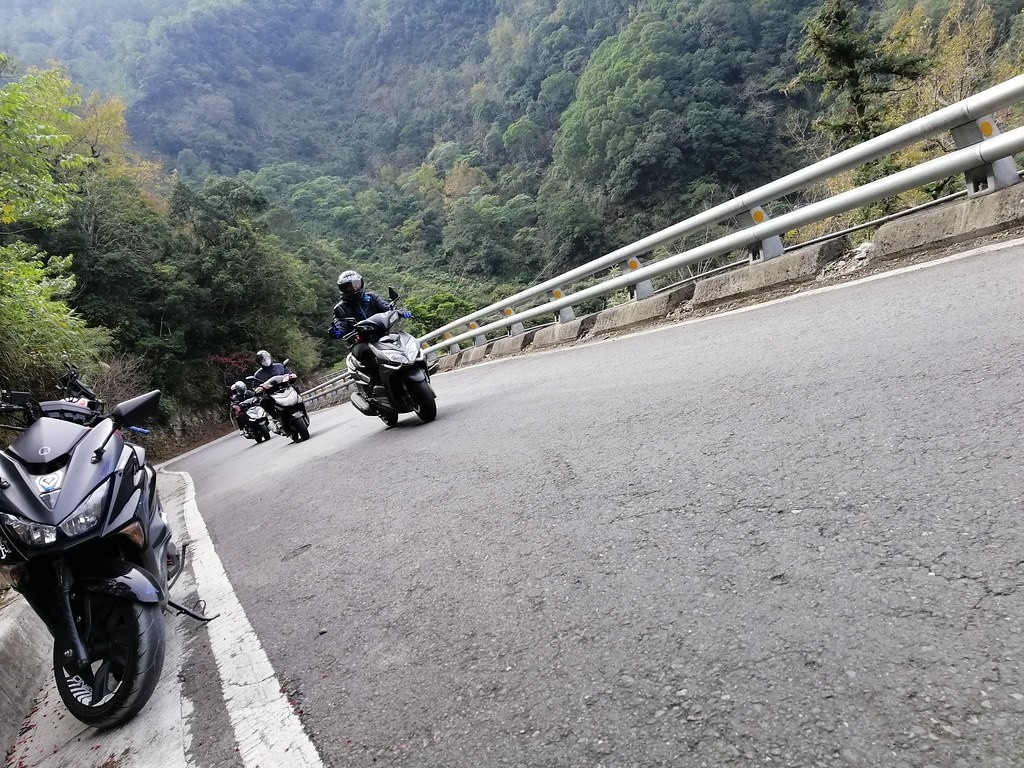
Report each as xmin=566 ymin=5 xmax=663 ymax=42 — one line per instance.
xmin=235 ymin=381 xmax=247 ymax=395
xmin=255 ymin=349 xmax=273 ymax=370
xmin=231 ymin=384 xmax=237 ymax=395
xmin=337 ymin=270 xmax=365 ymax=303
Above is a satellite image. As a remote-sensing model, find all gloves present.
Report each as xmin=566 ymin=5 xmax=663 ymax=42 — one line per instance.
xmin=396 ymin=307 xmax=412 ymax=319
xmin=289 ymin=374 xmax=298 ymax=379
xmin=255 ymin=386 xmax=263 ymax=395
xmin=328 ymin=326 xmax=342 ymax=339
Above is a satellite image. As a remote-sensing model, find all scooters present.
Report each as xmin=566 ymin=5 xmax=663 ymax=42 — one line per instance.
xmin=329 ymin=286 xmax=437 ymax=426
xmin=229 ymin=391 xmax=271 ymax=444
xmin=244 ymin=358 xmax=310 ymax=444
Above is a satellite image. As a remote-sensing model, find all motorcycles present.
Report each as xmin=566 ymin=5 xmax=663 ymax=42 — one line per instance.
xmin=0 ymin=380 xmax=221 ymax=730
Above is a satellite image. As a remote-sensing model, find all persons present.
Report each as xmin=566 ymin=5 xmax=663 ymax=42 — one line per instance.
xmin=252 ymin=350 xmax=303 ymax=424
xmin=230 ymin=381 xmax=268 ymax=436
xmin=333 ymin=270 xmax=439 ymax=398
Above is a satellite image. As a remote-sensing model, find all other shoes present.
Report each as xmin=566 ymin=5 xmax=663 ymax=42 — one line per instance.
xmin=428 ymin=359 xmax=440 ymax=376
xmin=276 ymin=422 xmax=282 ymax=429
xmin=372 ymin=386 xmax=386 ymax=398
xmin=239 ymin=430 xmax=244 ymax=436
